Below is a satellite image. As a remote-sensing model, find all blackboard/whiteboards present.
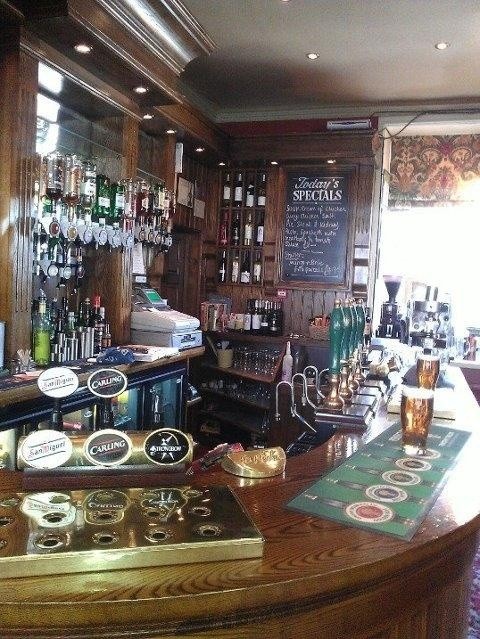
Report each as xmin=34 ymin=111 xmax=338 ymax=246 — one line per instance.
xmin=276 ymin=164 xmax=356 ymax=288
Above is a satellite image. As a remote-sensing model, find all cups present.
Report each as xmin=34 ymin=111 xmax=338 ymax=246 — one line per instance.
xmin=400 ymin=389 xmax=435 ymax=457
xmin=417 ymin=356 xmax=440 ymax=388
xmin=223 ymin=342 xmax=281 ymax=400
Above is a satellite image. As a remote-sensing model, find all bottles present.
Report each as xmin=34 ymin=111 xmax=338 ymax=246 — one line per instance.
xmin=242 ymin=297 xmax=284 ymax=333
xmin=219 ymin=170 xmax=268 ymax=283
xmin=113 ymin=175 xmax=176 ymax=255
xmin=34 ymin=152 xmax=113 ymax=366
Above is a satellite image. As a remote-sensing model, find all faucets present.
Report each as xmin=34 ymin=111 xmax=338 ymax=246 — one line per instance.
xmin=274 ymin=381 xmax=317 ymax=431
xmin=291 ymin=373 xmax=316 ymax=409
xmin=301 ymin=365 xmax=322 ymax=406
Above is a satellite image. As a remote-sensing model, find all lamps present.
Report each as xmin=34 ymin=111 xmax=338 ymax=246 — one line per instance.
xmin=326 ymin=119 xmax=372 ymax=130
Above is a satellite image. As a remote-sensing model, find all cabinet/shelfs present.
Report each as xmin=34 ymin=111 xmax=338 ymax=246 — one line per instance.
xmin=188 ymin=330 xmax=330 ymax=452
xmin=215 ymin=166 xmax=268 ymax=288
xmin=0 ymin=364 xmax=187 ymax=471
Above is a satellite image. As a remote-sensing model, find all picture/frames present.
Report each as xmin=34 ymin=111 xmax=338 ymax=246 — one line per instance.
xmin=176 ymin=172 xmax=195 ymax=209
